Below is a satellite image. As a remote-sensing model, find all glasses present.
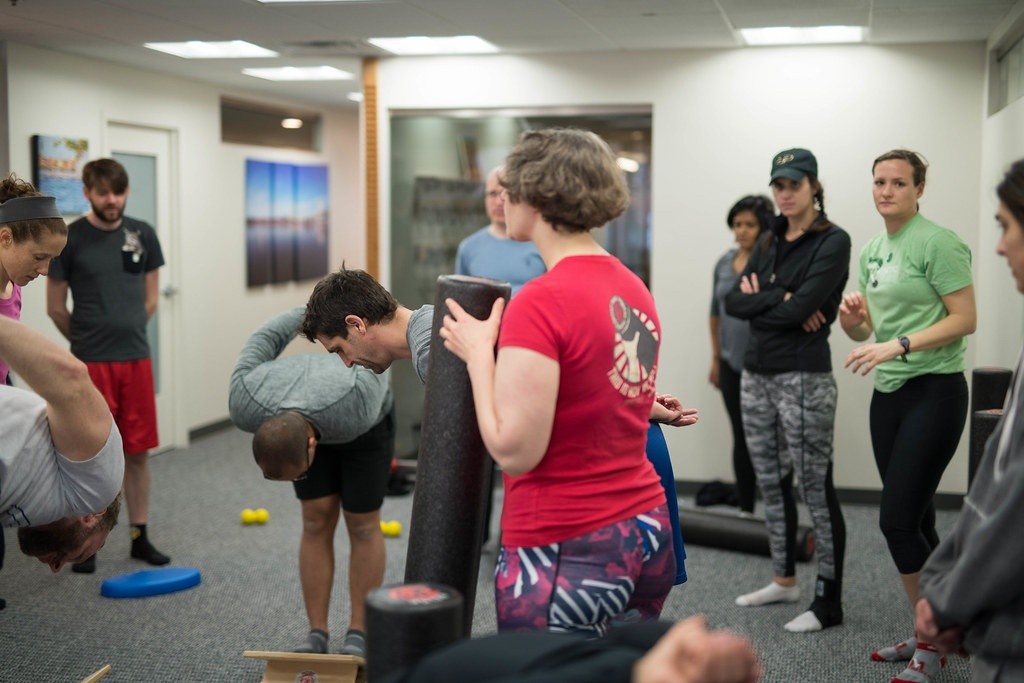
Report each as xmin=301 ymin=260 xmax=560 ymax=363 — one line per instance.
xmin=263 ymin=438 xmax=309 ymax=480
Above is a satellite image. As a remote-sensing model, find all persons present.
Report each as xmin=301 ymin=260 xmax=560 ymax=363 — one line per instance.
xmin=707 ymin=196 xmax=774 ymax=515
xmin=403 ymin=613 xmax=761 ymax=683
xmin=838 ymin=150 xmax=978 ymax=683
xmin=440 ymin=128 xmax=674 ymax=636
xmin=223 ymin=307 xmax=397 ymax=655
xmin=303 ymin=270 xmax=696 ymax=585
xmin=914 ymin=160 xmax=1024 ymax=683
xmin=46 ymin=159 xmax=170 ymax=571
xmin=0 ymin=176 xmax=124 ymax=610
xmin=722 ymin=148 xmax=850 ymax=634
xmin=0 ymin=314 xmax=126 ymax=575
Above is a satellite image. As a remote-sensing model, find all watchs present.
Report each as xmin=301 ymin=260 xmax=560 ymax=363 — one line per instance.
xmin=898 ymin=336 xmax=910 ymax=362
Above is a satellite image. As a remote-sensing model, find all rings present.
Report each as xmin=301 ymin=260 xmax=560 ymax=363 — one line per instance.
xmin=843 ymin=300 xmax=845 ymax=303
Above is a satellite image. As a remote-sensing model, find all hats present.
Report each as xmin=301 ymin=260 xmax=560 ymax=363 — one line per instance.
xmin=769 ymin=149 xmax=818 ymax=187
